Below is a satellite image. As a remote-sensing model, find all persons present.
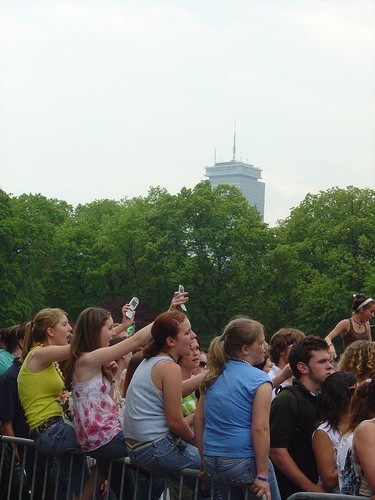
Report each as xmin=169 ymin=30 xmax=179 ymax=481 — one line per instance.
xmin=269 ymin=335 xmax=333 ymax=493
xmin=194 ymin=314 xmax=282 ymax=500
xmin=0 ymin=320 xmax=375 ymax=500
xmin=17 ymin=304 xmax=136 ymax=500
xmin=324 ymin=295 xmax=375 ymax=361
xmin=63 ymin=291 xmax=189 ymax=500
xmin=123 ymin=310 xmax=210 ymax=476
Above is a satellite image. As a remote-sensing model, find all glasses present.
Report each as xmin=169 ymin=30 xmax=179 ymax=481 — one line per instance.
xmin=199 ymin=361 xmax=207 ymax=367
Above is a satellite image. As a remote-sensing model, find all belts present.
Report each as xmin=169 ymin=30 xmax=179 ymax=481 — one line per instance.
xmin=32 ymin=416 xmax=63 ymax=439
xmin=125 ymin=436 xmax=166 ymax=453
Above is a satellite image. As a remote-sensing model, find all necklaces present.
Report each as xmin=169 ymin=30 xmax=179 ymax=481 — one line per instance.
xmin=353 ymin=317 xmax=363 ymax=328
xmin=162 ymin=350 xmax=177 ymax=364
xmin=36 ymin=342 xmax=60 ymax=369
xmin=240 ymin=359 xmax=253 ymax=367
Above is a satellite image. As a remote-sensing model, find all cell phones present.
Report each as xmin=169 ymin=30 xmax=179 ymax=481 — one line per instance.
xmin=179 ymin=285 xmax=187 ymax=311
xmin=126 ymin=297 xmax=139 ymax=318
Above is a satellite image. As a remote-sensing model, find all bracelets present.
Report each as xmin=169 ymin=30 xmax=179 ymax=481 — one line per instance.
xmin=255 ymin=474 xmax=270 ymax=482
xmin=328 ymin=343 xmax=332 ymax=347
